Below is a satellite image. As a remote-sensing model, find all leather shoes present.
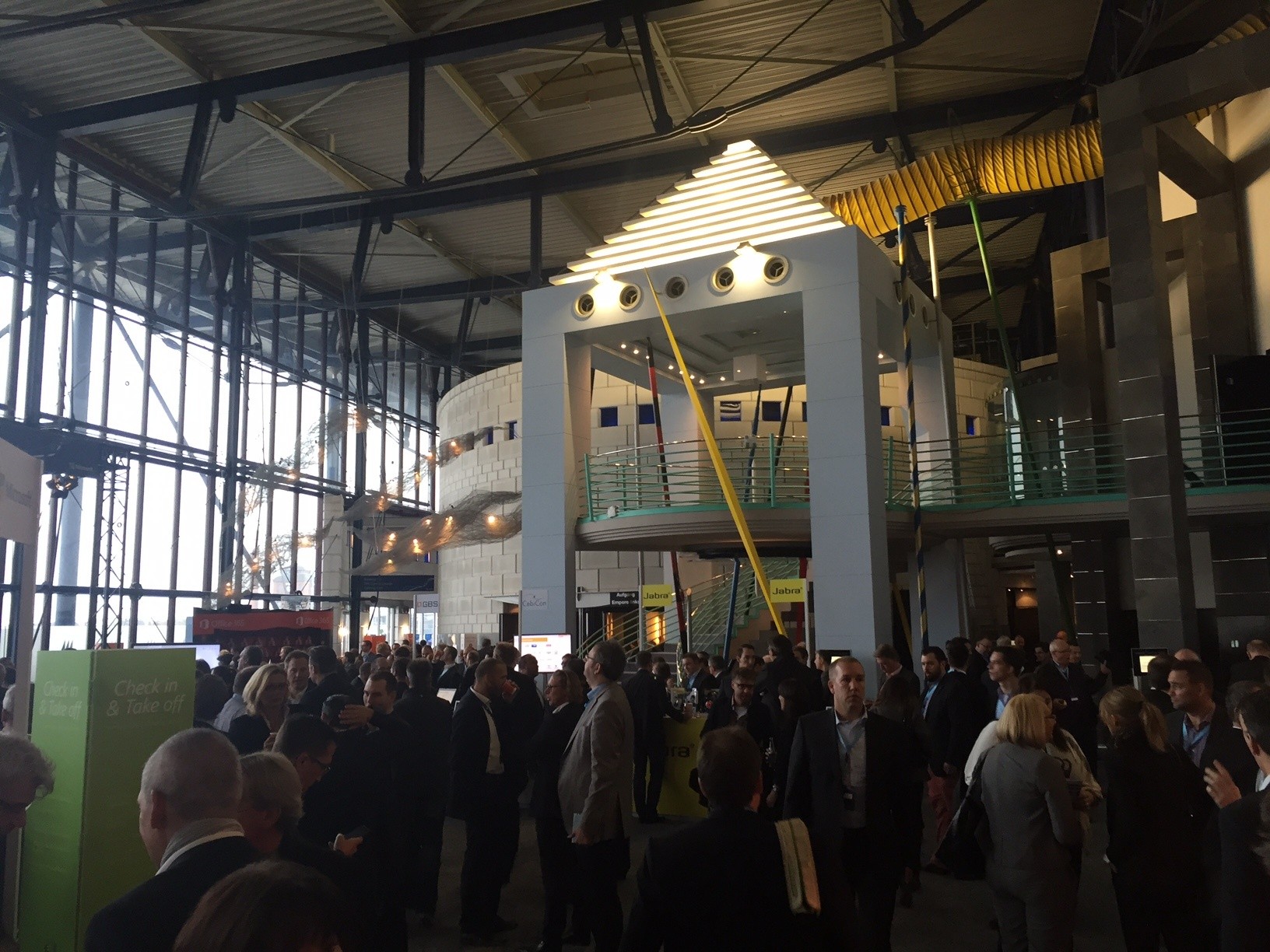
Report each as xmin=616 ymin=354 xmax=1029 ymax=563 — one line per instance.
xmin=459 ymin=928 xmax=506 ymax=941
xmin=488 ymin=913 xmax=517 ymax=935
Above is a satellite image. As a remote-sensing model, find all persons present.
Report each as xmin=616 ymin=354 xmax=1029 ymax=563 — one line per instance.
xmin=0 ymin=628 xmax=1269 ymax=952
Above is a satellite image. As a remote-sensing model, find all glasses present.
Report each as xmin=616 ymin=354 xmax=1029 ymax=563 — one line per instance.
xmin=264 ymin=682 xmax=289 ymax=692
xmin=733 ymin=680 xmax=754 ymax=690
xmin=312 ymin=755 xmax=331 ymax=774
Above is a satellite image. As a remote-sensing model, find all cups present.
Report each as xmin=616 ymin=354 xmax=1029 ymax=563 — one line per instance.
xmin=684 ymin=703 xmax=693 ymax=717
xmin=501 ymin=681 xmax=517 ymax=697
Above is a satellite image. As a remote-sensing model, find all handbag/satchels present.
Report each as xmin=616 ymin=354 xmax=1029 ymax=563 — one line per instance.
xmin=925 ymin=745 xmax=997 ymax=880
